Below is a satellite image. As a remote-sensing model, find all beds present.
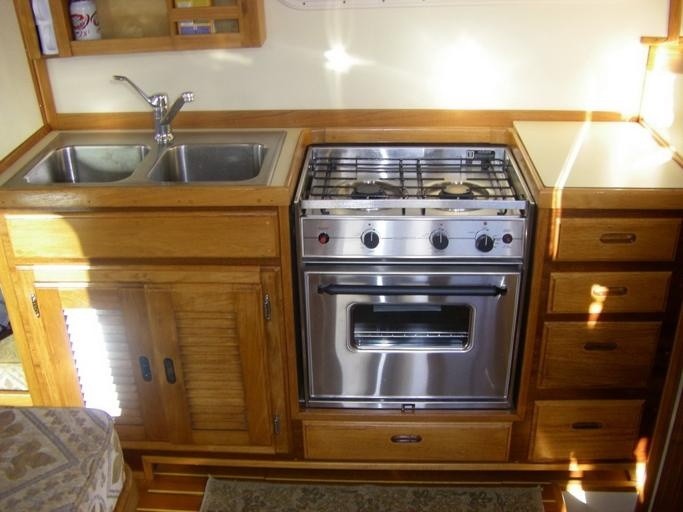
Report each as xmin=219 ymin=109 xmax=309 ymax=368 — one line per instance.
xmin=0 ymin=405 xmax=139 ymax=512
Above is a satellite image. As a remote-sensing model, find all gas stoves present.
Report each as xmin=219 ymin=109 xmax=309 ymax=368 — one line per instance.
xmin=305 ymin=148 xmax=526 ymax=262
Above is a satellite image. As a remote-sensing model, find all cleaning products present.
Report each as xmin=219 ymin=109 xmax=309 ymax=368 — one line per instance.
xmin=33 ymin=0 xmax=59 ymax=57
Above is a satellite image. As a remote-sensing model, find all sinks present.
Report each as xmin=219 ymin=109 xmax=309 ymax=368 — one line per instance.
xmin=141 ymin=131 xmax=288 ymax=186
xmin=0 ymin=129 xmax=155 ymax=187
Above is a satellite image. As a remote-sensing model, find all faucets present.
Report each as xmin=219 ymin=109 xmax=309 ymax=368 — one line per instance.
xmin=113 ymin=74 xmax=194 ymax=144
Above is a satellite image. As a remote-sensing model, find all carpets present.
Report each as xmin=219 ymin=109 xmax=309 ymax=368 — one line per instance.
xmin=199 ymin=473 xmax=546 ymax=512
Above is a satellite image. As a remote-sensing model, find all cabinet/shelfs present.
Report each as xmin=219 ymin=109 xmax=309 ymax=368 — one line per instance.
xmin=516 ymin=189 xmax=683 ymax=471
xmin=0 ymin=188 xmax=294 ymax=454
xmin=12 ymin=0 xmax=267 ymax=60
xmin=294 ymin=418 xmax=522 ymax=470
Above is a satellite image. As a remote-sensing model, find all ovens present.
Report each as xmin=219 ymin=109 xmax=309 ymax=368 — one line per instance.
xmin=300 ymin=264 xmax=524 ymax=408
